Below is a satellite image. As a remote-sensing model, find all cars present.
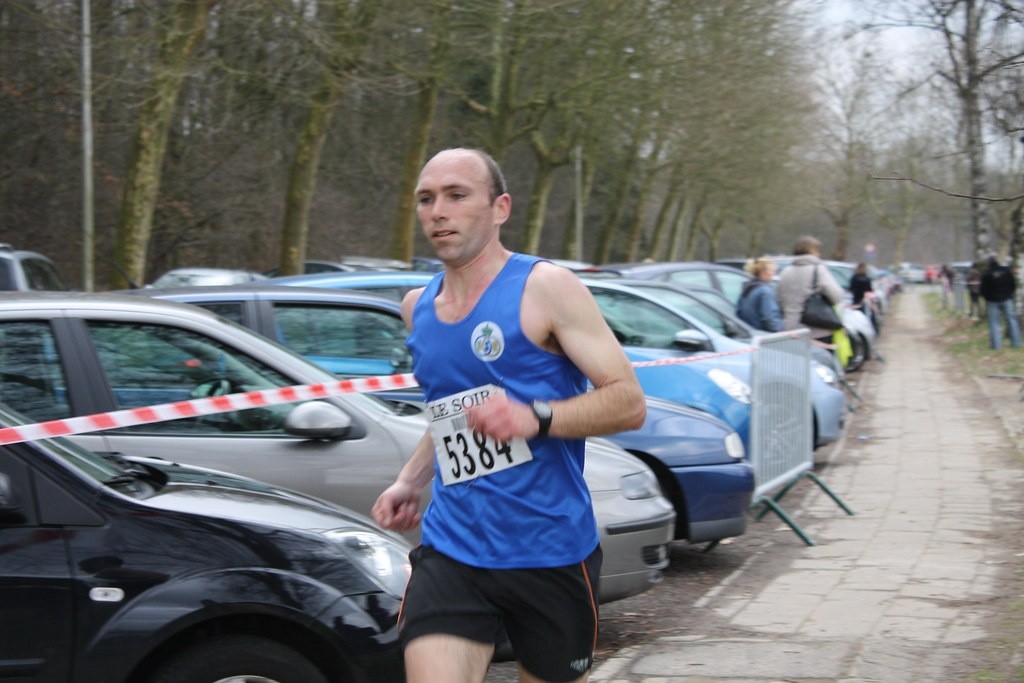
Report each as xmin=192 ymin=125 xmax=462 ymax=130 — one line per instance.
xmin=45 ymin=281 xmax=756 ymax=544
xmin=0 ymin=399 xmax=414 ymax=683
xmin=133 ymin=252 xmax=974 ymax=462
xmin=0 ymin=285 xmax=679 ymax=663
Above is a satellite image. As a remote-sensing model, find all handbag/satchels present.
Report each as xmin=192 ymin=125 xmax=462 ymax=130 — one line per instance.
xmin=801 ymin=265 xmax=843 ymax=330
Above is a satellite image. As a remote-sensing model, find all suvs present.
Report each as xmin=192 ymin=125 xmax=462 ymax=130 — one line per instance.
xmin=0 ymin=241 xmax=70 ymax=292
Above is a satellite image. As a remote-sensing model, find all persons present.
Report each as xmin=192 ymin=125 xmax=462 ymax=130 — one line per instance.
xmin=372 ymin=147 xmax=650 ymax=683
xmin=734 ymin=236 xmax=1024 ymax=372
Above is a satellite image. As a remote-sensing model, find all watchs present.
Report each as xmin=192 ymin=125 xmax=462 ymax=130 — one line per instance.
xmin=529 ymin=398 xmax=555 ymax=439
